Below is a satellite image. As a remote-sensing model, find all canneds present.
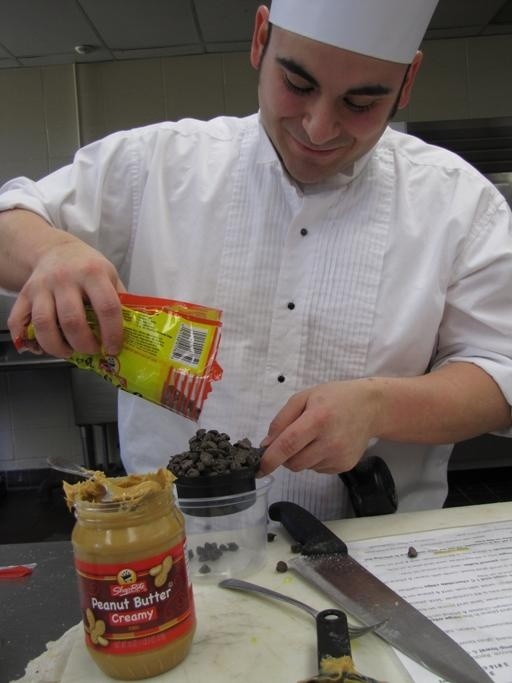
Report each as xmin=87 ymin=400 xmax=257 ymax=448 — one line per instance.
xmin=71 ymin=473 xmax=196 ymax=681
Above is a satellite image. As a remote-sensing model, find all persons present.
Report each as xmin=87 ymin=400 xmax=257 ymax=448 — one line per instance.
xmin=0 ymin=0 xmax=512 ymax=535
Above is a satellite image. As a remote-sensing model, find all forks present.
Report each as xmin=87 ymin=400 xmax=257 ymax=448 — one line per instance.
xmin=219 ymin=579 xmax=390 ymax=640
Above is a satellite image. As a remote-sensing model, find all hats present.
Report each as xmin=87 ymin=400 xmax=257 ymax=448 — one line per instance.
xmin=269 ymin=0 xmax=438 ymax=66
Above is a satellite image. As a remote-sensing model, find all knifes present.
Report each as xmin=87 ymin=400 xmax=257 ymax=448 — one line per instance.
xmin=268 ymin=501 xmax=493 ymax=683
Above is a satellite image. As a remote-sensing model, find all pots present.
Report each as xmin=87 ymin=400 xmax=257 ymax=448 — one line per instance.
xmin=299 ymin=608 xmax=378 ymax=683
xmin=170 ymin=443 xmax=268 ymax=517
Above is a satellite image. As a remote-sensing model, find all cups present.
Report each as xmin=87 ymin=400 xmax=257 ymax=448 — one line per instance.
xmin=165 ymin=474 xmax=275 ymax=586
